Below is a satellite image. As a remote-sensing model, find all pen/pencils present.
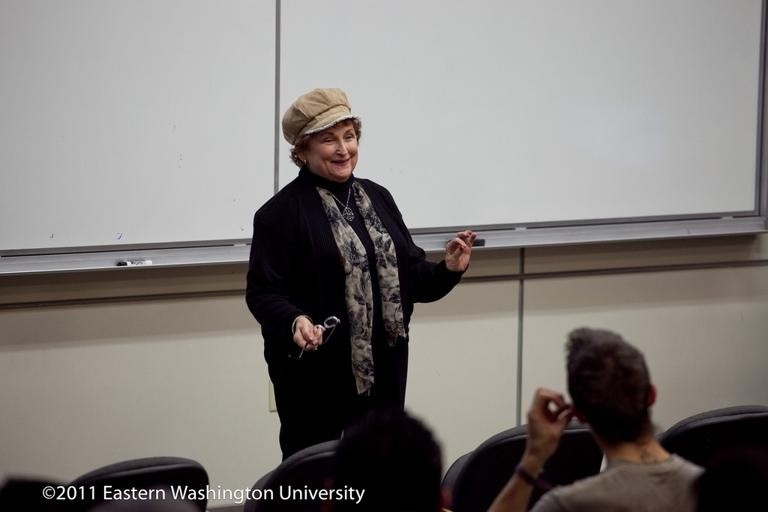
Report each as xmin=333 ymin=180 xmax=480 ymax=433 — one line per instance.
xmin=117 ymin=259 xmax=153 ymax=266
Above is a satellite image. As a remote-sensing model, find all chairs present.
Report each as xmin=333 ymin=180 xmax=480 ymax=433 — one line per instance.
xmin=69 ymin=401 xmax=768 ymax=512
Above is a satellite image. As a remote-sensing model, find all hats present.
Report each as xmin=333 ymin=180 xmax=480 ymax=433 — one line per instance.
xmin=281 ymin=85 xmax=359 ymax=147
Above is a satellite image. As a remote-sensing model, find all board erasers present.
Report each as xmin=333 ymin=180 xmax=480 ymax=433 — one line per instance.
xmin=471 ymin=239 xmax=485 ymax=246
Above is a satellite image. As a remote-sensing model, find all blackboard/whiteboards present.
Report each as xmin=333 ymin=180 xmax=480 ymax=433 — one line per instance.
xmin=0 ymin=0 xmax=768 ymax=277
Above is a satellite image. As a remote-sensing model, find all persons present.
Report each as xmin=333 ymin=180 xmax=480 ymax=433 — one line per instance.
xmin=486 ymin=328 xmax=707 ymax=512
xmin=688 ymin=451 xmax=768 ymax=511
xmin=322 ymin=408 xmax=444 ymax=512
xmin=244 ymin=89 xmax=477 ymax=463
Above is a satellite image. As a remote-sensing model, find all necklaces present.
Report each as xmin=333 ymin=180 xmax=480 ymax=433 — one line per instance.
xmin=328 ymin=188 xmax=355 ymax=221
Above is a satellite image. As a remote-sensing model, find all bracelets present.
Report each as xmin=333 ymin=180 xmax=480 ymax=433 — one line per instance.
xmin=291 ymin=315 xmax=313 ymax=335
xmin=515 ymin=465 xmax=537 ymax=486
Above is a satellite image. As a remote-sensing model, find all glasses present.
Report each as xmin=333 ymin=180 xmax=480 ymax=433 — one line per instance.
xmin=299 ymin=316 xmax=341 ymax=359
